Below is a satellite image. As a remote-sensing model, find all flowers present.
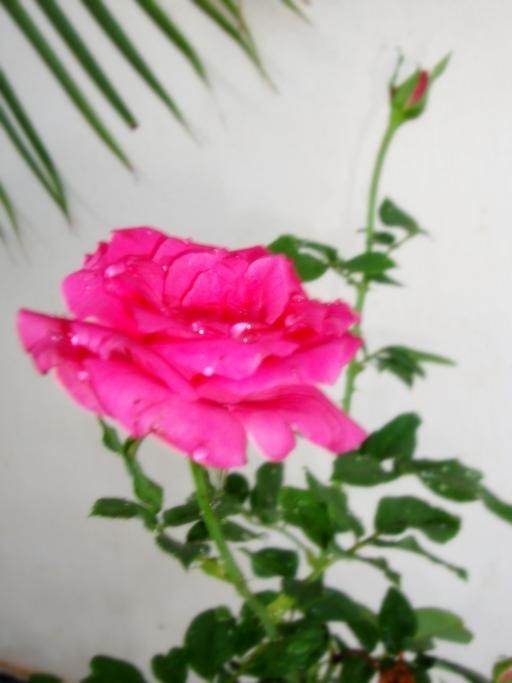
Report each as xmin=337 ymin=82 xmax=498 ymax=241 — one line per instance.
xmin=13 ymin=48 xmax=512 ymax=682
